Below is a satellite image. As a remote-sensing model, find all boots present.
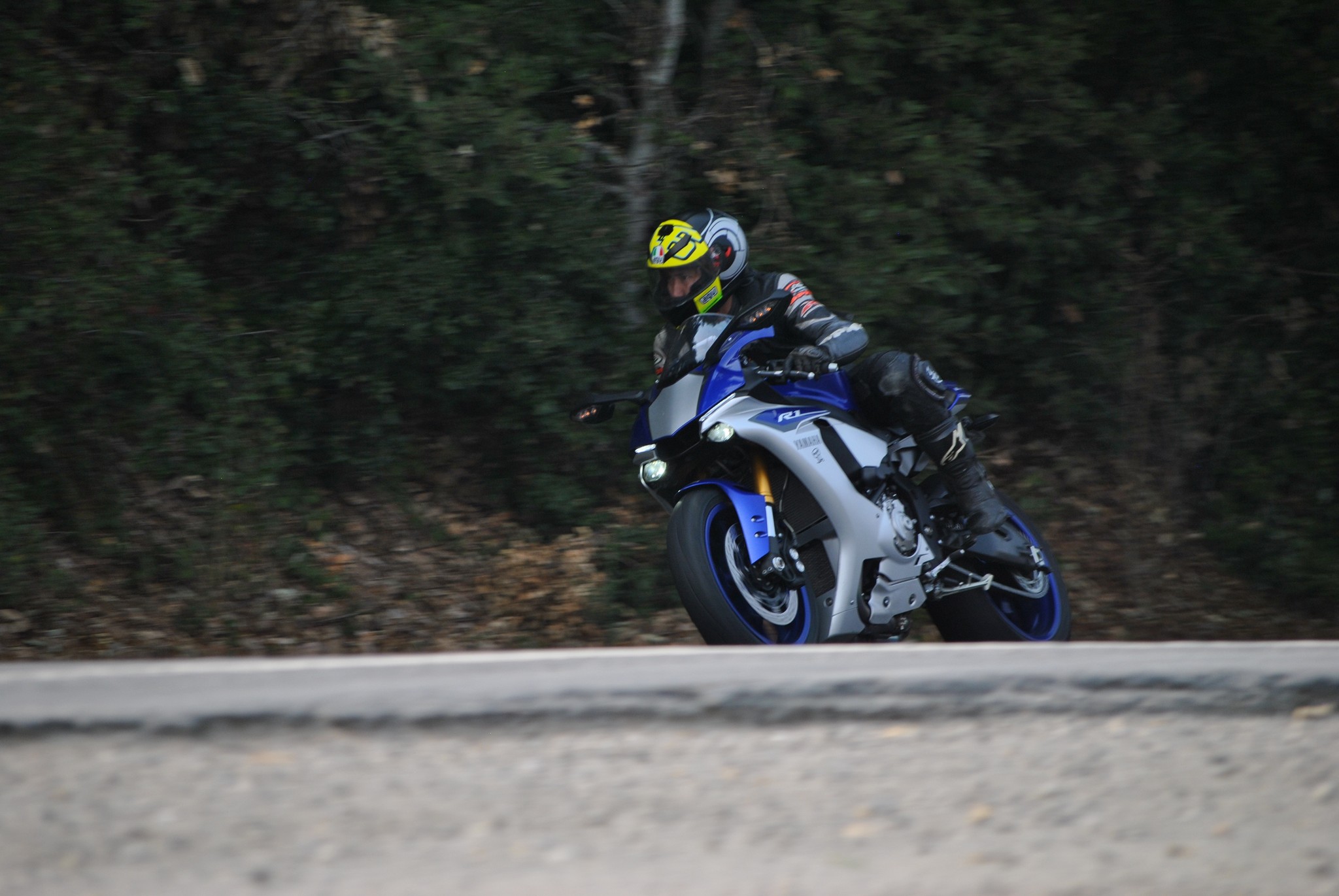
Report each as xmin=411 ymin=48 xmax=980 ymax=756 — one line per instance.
xmin=946 ymin=458 xmax=1015 ymax=535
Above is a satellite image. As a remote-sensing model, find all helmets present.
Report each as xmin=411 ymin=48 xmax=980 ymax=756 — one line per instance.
xmin=645 ymin=206 xmax=750 ymax=326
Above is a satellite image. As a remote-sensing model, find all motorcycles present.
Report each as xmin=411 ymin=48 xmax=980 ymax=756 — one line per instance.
xmin=567 ymin=287 xmax=1066 ymax=645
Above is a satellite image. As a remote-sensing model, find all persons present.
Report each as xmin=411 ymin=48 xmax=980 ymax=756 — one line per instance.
xmin=645 ymin=205 xmax=1011 ymax=533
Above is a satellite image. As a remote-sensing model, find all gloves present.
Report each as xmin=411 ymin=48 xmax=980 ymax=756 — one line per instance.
xmin=786 ymin=343 xmax=831 ymax=379
